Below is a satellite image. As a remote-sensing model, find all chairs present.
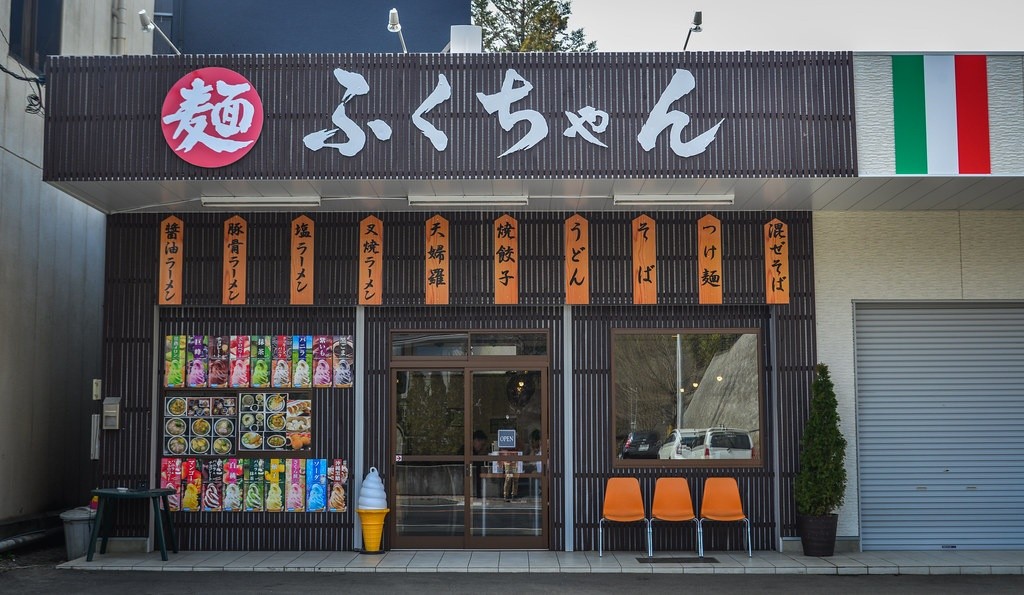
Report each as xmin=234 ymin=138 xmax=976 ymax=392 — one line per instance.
xmin=599 ymin=477 xmax=650 ymax=557
xmin=650 ymin=476 xmax=702 ymax=557
xmin=700 ymin=477 xmax=753 ymax=558
xmin=444 ymin=465 xmax=490 ymax=532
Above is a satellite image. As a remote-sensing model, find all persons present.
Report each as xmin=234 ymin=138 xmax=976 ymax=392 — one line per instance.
xmin=456 ymin=431 xmax=488 ymax=499
xmin=527 ymin=429 xmax=542 ymax=473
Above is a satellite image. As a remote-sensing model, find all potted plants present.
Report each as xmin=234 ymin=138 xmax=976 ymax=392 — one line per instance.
xmin=796 ymin=363 xmax=850 ymax=558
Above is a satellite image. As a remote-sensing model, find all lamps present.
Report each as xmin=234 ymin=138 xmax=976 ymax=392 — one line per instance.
xmin=613 ymin=193 xmax=735 ymax=206
xmin=138 ymin=7 xmax=181 ymax=57
xmin=387 ymin=8 xmax=409 ymax=53
xmin=407 ymin=194 xmax=528 ymax=206
xmin=201 ymin=196 xmax=324 ymax=210
xmin=683 ymin=11 xmax=703 ymax=52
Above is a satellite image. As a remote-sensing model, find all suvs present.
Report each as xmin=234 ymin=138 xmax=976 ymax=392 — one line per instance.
xmin=657 ymin=429 xmax=708 ymax=458
xmin=622 ymin=430 xmax=661 ymax=459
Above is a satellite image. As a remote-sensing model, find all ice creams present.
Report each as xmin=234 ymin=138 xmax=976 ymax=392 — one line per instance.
xmin=356 ymin=466 xmax=390 ymax=548
xmin=161 ymin=484 xmax=345 ymax=511
xmin=167 ymin=360 xmax=352 ymax=388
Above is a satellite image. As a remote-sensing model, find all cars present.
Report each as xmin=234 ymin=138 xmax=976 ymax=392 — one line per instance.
xmin=690 ymin=425 xmax=756 ymax=460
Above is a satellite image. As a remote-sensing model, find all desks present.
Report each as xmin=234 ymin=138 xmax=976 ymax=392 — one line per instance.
xmin=482 ymin=470 xmax=542 ymax=537
xmin=85 ymin=484 xmax=179 ymax=565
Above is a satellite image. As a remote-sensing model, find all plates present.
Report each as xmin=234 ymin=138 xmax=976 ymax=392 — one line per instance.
xmin=167 ymin=397 xmax=287 ymax=456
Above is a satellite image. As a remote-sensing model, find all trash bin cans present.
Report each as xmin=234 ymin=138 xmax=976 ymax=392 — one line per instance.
xmin=60 ymin=507 xmax=97 ymax=562
